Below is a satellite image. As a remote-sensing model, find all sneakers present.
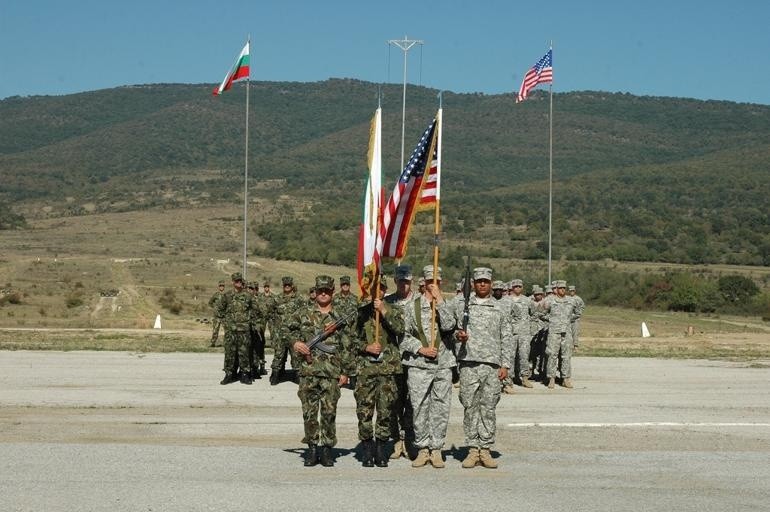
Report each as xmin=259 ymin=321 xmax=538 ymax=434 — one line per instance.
xmin=462 ymin=448 xmax=497 ymax=468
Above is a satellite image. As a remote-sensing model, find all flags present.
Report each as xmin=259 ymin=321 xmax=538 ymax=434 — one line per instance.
xmin=213 ymin=40 xmax=250 ymax=98
xmin=514 ymin=50 xmax=554 ymax=104
xmin=357 ymin=107 xmax=384 ymax=295
xmin=372 ymin=107 xmax=442 ymax=262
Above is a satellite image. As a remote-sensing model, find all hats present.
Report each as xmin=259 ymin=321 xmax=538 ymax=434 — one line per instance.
xmin=310 ymin=276 xmax=335 ymax=293
xmin=455 ymin=277 xmax=473 ymax=291
xmin=491 ymin=279 xmax=575 ymax=294
xmin=219 ymin=273 xmax=270 ymax=288
xmin=394 ymin=265 xmax=413 ymax=281
xmin=340 ymin=276 xmax=350 ymax=286
xmin=418 ymin=264 xmax=442 ymax=285
xmin=282 ymin=277 xmax=297 ymax=292
xmin=370 ymin=277 xmax=387 ymax=288
xmin=474 ymin=267 xmax=492 ymax=280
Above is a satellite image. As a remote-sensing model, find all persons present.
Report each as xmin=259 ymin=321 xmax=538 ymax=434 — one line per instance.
xmin=342 ymin=265 xmax=456 ymax=469
xmin=209 ymin=273 xmax=358 ymax=390
xmin=279 ymin=275 xmax=355 ymax=467
xmin=448 ymin=268 xmax=513 ymax=467
xmin=452 ymin=278 xmax=585 ymax=394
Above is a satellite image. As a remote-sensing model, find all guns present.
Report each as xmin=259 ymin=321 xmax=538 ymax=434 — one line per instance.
xmin=462 ymin=255 xmax=472 ymax=332
xmin=305 ymin=310 xmax=356 ymax=364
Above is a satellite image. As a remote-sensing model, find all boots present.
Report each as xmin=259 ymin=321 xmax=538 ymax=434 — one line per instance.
xmin=319 ymin=445 xmax=335 ymax=466
xmin=362 ymin=438 xmax=446 ymax=468
xmin=270 ymin=369 xmax=285 ymax=385
xmin=504 ymin=377 xmax=575 ymax=393
xmin=220 ymin=362 xmax=264 ymax=384
xmin=304 ymin=444 xmax=319 ymax=466
